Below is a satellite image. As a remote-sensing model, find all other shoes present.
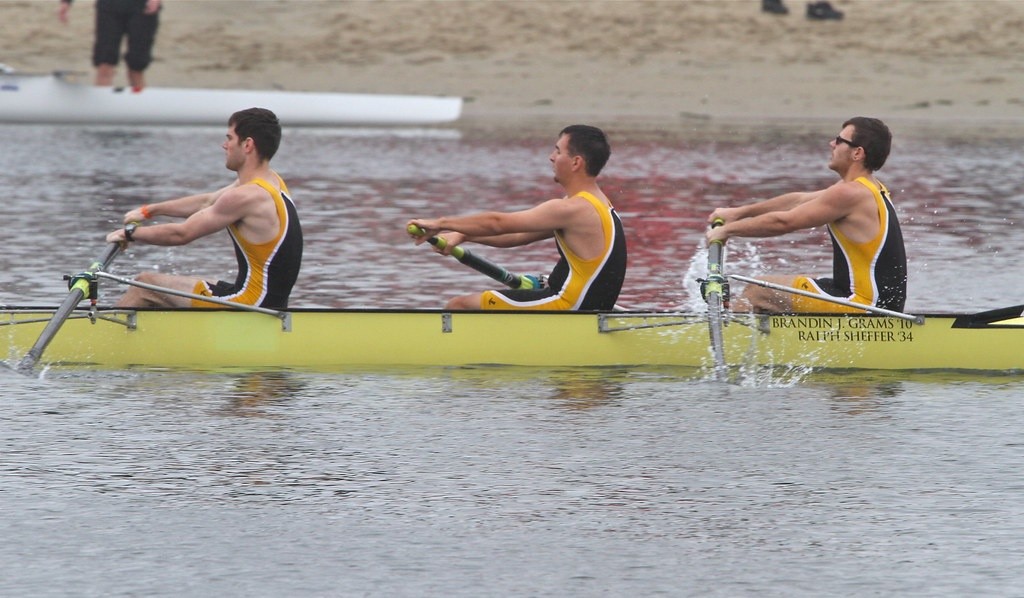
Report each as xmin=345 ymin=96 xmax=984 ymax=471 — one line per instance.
xmin=761 ymin=0 xmax=788 ymax=14
xmin=807 ymin=2 xmax=845 ymax=20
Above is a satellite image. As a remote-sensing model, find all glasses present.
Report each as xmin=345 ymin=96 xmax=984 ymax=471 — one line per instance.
xmin=835 ymin=135 xmax=867 ymax=155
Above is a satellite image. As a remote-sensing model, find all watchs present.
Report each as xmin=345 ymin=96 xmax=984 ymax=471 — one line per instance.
xmin=125 ymin=225 xmax=136 ymax=241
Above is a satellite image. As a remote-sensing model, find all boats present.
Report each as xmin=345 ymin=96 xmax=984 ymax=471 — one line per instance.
xmin=1 ymin=60 xmax=465 ymax=136
xmin=2 ymin=299 xmax=1024 ymax=379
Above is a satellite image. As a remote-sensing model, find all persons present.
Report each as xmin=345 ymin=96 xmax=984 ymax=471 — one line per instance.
xmin=704 ymin=116 xmax=908 ymax=315
xmin=106 ymin=107 xmax=303 ymax=311
xmin=761 ymin=0 xmax=845 ymax=20
xmin=58 ymin=0 xmax=162 ymax=87
xmin=405 ymin=124 xmax=628 ymax=313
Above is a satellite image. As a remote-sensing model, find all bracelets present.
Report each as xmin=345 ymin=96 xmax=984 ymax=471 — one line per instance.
xmin=141 ymin=204 xmax=151 ymax=219
